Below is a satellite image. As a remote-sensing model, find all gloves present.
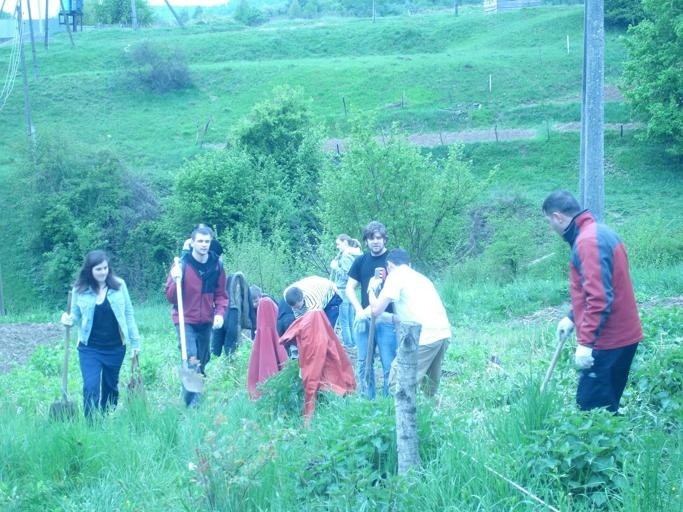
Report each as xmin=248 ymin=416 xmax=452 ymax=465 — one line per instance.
xmin=130 ymin=349 xmax=140 ymax=360
xmin=368 ymin=270 xmax=386 ymax=292
xmin=330 ymin=260 xmax=340 ymax=269
xmin=575 ymin=346 xmax=594 ymax=369
xmin=557 ymin=317 xmax=573 ymax=340
xmin=61 ymin=313 xmax=73 ymax=326
xmin=170 ymin=267 xmax=183 ymax=282
xmin=212 ymin=314 xmax=223 ymax=330
xmin=354 ymin=314 xmax=367 ymax=332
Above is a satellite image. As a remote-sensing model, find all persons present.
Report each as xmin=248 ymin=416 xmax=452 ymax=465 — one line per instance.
xmin=366 ymin=246 xmax=451 ymax=400
xmin=248 ymin=284 xmax=276 ymax=339
xmin=163 ymin=228 xmax=230 ymax=404
xmin=276 ymin=298 xmax=298 ymax=360
xmin=284 ymin=275 xmax=344 ymax=333
xmin=183 ymin=223 xmax=224 ymax=260
xmin=543 ymin=190 xmax=643 ymax=417
xmin=61 ymin=249 xmax=141 ymax=425
xmin=344 ymin=221 xmax=397 ymax=401
xmin=329 ymin=234 xmax=365 ymax=349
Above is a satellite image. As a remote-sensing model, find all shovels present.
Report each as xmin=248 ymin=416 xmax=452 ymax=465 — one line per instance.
xmin=173 ymin=257 xmax=205 ymax=393
xmin=49 ymin=284 xmax=79 ymax=423
